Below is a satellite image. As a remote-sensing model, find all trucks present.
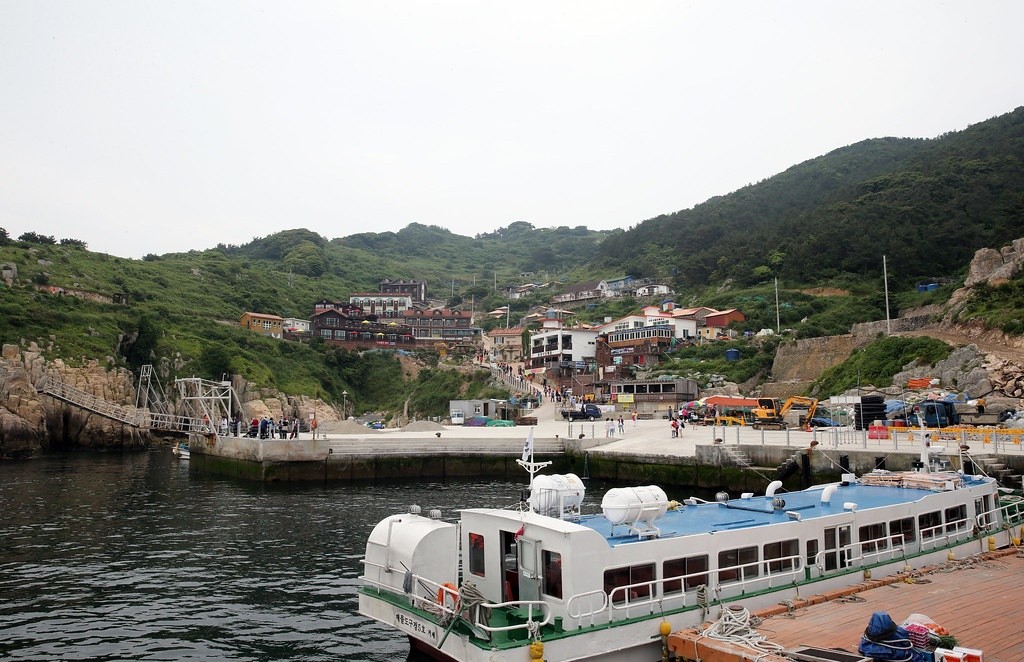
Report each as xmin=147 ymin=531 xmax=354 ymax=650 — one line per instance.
xmin=561 ymin=404 xmax=602 ymax=421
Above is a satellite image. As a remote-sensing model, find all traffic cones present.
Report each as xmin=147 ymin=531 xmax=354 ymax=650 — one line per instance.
xmin=807 ymin=423 xmax=814 ymax=432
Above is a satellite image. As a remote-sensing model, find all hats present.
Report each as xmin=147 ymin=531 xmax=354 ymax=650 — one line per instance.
xmin=284 ymin=416 xmax=288 ymax=419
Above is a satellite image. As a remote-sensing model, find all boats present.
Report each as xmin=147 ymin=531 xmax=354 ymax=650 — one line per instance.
xmin=353 ymin=470 xmax=1024 ymax=662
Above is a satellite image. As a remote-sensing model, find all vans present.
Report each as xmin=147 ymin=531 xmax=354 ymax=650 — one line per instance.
xmin=907 ymin=402 xmax=947 ymax=429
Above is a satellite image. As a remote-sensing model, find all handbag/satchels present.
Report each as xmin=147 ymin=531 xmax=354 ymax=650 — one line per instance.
xmin=680 ymin=422 xmax=685 ymax=428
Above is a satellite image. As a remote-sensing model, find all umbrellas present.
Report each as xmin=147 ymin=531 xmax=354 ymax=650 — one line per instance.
xmin=362 ymin=320 xmax=370 ymax=323
xmin=699 ymin=397 xmax=714 ymax=405
xmin=377 ymin=333 xmax=385 ymax=335
xmin=405 ymin=334 xmax=410 ymax=336
xmin=387 ymin=321 xmax=397 ymax=326
xmin=288 ymin=328 xmax=297 ymax=331
xmin=297 ymin=329 xmax=304 ymax=331
xmin=351 ymin=330 xmax=358 ymax=334
xmin=682 ymin=401 xmax=705 ymax=407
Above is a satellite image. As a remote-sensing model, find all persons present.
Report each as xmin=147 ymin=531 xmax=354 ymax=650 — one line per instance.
xmin=221 ymin=407 xmax=241 ymax=437
xmin=517 ymin=365 xmax=535 ymax=384
xmin=617 ymin=414 xmax=624 ymax=435
xmin=201 ymin=414 xmax=209 ymax=431
xmin=491 ymin=359 xmax=512 ymax=375
xmin=243 ymin=414 xmax=299 ymax=439
xmin=678 ymin=406 xmax=754 ymax=430
xmin=542 ymin=377 xmax=591 ymax=407
xmin=667 ymin=405 xmax=672 ymax=421
xmin=631 ymin=408 xmax=637 ymax=426
xmin=477 ymin=342 xmax=491 ymax=365
xmin=605 ymin=418 xmax=615 ymax=437
xmin=976 ymin=396 xmax=987 ymax=413
xmin=671 ymin=417 xmax=684 ymax=438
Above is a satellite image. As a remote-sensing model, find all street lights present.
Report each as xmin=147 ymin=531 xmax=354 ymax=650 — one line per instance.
xmin=341 ymin=389 xmax=348 ymax=421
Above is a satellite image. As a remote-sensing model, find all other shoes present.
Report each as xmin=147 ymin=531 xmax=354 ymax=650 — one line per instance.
xmin=622 ymin=432 xmax=625 ymax=435
xmin=610 ymin=435 xmax=611 ymax=438
xmin=553 ymin=400 xmax=555 ymax=403
xmin=226 ymin=434 xmax=228 ymax=437
xmin=233 ymin=435 xmax=237 ymax=437
xmin=675 ymin=436 xmax=678 ymax=437
xmin=619 ymin=432 xmax=621 ymax=435
xmin=612 ymin=436 xmax=614 ymax=439
xmin=221 ymin=434 xmax=223 ymax=436
xmin=669 ymin=420 xmax=671 ymax=421
xmin=684 ymin=421 xmax=687 ymax=423
xmin=550 ymin=400 xmax=552 ymax=403
xmin=273 ymin=437 xmax=276 ymax=440
xmin=672 ymin=436 xmax=675 ymax=438
xmin=606 ymin=435 xmax=609 ymax=439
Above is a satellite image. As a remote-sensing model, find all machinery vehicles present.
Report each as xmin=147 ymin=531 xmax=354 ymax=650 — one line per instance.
xmin=750 ymin=395 xmax=820 ymax=429
